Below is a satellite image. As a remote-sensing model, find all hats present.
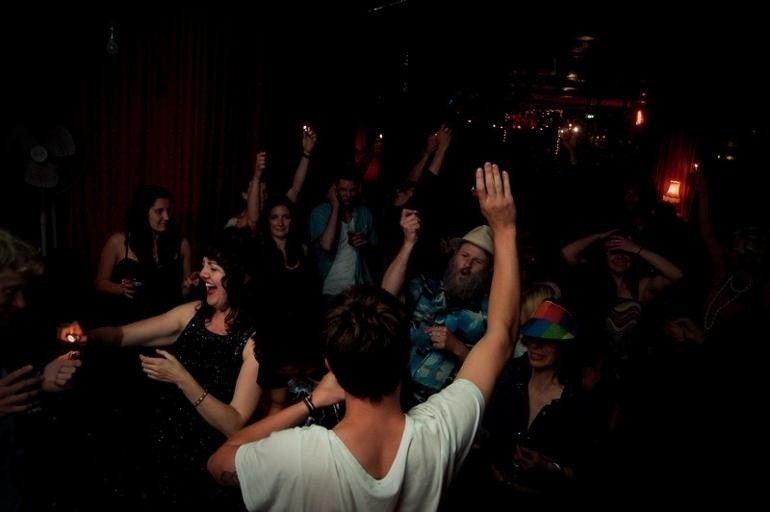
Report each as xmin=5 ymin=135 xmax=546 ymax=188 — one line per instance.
xmin=520 ymin=298 xmax=574 ymax=340
xmin=460 ymin=224 xmax=493 ymax=255
